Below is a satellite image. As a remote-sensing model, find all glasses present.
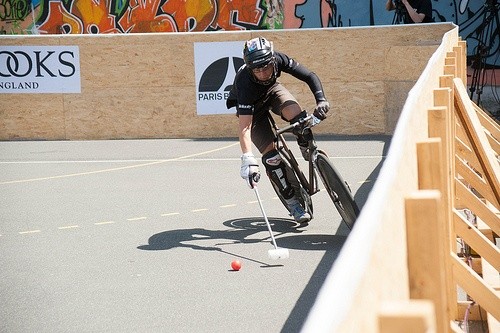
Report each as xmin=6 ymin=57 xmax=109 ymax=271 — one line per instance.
xmin=251 ymin=63 xmax=273 ymax=73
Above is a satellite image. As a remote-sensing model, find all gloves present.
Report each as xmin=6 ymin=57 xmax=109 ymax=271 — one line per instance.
xmin=240 ymin=152 xmax=261 ymax=189
xmin=314 ymin=100 xmax=330 ymax=120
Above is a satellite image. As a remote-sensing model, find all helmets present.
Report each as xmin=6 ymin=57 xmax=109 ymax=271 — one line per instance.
xmin=243 ymin=37 xmax=277 ymax=85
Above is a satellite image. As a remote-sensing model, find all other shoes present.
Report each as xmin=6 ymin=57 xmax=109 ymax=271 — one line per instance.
xmin=288 ymin=199 xmax=311 ymax=223
xmin=299 ymin=146 xmax=315 ymax=161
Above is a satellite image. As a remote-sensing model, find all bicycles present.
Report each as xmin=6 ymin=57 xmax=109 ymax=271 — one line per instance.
xmin=262 ymin=106 xmax=361 ymax=231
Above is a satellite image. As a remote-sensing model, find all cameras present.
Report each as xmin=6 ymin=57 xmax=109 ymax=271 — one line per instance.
xmin=485 ymin=0 xmax=500 ymax=6
xmin=394 ymin=0 xmax=406 ymax=9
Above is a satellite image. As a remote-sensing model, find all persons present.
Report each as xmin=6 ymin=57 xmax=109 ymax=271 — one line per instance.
xmin=386 ymin=0 xmax=432 ymax=24
xmin=226 ymin=36 xmax=330 ymax=224
